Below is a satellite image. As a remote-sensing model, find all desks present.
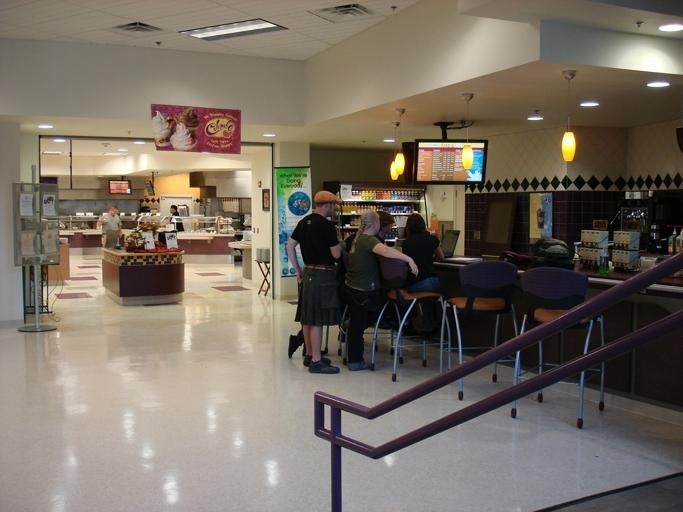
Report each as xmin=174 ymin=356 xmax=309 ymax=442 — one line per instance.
xmin=228 ymin=240 xmax=252 ymax=280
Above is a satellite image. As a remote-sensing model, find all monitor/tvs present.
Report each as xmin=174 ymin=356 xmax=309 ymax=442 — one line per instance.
xmin=414 ymin=139 xmax=488 ymax=185
xmin=108 ymin=179 xmax=132 ymax=195
xmin=402 ymin=142 xmax=415 ymax=186
xmin=145 ymin=180 xmax=155 ymax=195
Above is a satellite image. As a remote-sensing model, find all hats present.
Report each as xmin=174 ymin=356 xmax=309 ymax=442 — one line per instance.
xmin=314 ymin=190 xmax=340 ymax=202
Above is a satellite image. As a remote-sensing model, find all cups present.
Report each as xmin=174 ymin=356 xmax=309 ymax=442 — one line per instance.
xmin=60 ymin=238 xmax=67 ymax=243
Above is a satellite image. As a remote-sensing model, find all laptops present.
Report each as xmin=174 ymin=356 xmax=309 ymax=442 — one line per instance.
xmin=441 ymin=230 xmax=460 ymax=258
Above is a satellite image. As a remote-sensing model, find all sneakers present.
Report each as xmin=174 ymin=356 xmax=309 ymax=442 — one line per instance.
xmin=287 ymin=334 xmax=339 ymax=373
xmin=348 ymin=359 xmax=369 ymax=371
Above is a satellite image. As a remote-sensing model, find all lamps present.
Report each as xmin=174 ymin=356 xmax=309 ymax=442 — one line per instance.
xmin=461 ymin=93 xmax=475 ymax=171
xmin=390 ymin=108 xmax=405 ymax=181
xmin=561 ymin=69 xmax=577 ymax=162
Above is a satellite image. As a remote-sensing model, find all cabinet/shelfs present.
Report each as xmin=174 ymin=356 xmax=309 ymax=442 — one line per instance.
xmin=42 ymin=243 xmax=70 ymax=281
xmin=322 ymin=180 xmax=427 ymax=241
xmin=102 ymin=241 xmax=185 ymax=306
xmin=58 ymin=216 xmax=235 ymax=255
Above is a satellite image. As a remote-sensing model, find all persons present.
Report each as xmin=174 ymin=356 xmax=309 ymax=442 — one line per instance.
xmin=286 ymin=190 xmax=340 ymax=375
xmin=342 ymin=209 xmax=419 ymax=372
xmin=98 ymin=206 xmax=123 ymax=248
xmin=169 ymin=206 xmax=184 ymax=230
xmin=287 ymin=211 xmax=397 ymax=360
xmin=402 ymin=213 xmax=445 ymax=294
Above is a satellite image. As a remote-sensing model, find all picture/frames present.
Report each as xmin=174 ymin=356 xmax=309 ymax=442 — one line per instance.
xmin=262 ymin=188 xmax=270 ymax=211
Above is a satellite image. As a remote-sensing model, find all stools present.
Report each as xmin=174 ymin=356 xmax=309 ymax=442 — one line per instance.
xmin=253 ymin=260 xmax=270 ymax=296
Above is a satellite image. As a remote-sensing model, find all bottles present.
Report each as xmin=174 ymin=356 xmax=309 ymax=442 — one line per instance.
xmin=350 ymin=216 xmax=396 ymax=227
xmin=431 ymin=213 xmax=438 ymax=232
xmin=668 ymin=228 xmax=678 ymax=254
xmin=648 ymin=224 xmax=661 ymax=252
xmin=351 ymin=191 xmax=422 ymax=200
xmin=343 ymin=229 xmax=398 ymax=239
xmin=341 ymin=201 xmax=414 ymax=214
xmin=598 ymin=247 xmax=610 ymax=275
xmin=676 ymin=229 xmax=683 ymax=254
